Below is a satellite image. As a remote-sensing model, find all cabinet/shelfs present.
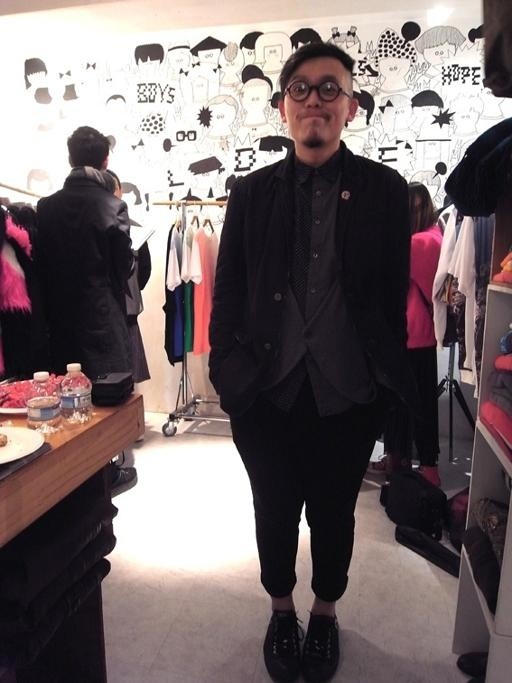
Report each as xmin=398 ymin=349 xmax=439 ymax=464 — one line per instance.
xmin=451 ymin=207 xmax=512 ymax=681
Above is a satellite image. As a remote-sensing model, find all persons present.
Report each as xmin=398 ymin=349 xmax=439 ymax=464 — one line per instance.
xmin=104 ymin=167 xmax=153 ymax=446
xmin=364 ymin=180 xmax=445 ymax=491
xmin=33 ymin=125 xmax=136 ymax=495
xmin=206 ymin=41 xmax=410 ymax=683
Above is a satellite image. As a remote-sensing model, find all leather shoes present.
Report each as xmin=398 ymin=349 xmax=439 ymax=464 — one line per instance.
xmin=111 ymin=467 xmax=136 ymax=490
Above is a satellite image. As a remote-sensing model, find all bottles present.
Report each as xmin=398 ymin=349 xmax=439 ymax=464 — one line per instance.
xmin=26 ymin=370 xmax=61 ymax=435
xmin=61 ymin=363 xmax=96 ymax=424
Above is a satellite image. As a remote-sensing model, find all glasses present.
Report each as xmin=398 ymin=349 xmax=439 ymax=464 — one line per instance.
xmin=283 ymin=81 xmax=353 ymax=101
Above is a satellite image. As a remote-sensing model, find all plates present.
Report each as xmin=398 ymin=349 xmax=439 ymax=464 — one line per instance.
xmin=1 ymin=426 xmax=45 ymax=464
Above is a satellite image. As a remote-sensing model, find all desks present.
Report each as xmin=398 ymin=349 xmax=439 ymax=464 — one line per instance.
xmin=2 ymin=379 xmax=144 ymax=682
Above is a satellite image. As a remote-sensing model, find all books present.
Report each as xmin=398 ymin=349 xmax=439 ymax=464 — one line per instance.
xmin=128 ymin=223 xmax=154 ymax=251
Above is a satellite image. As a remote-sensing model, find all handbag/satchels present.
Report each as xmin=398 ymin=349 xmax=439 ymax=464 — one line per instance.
xmin=386 ymin=471 xmax=447 ymax=535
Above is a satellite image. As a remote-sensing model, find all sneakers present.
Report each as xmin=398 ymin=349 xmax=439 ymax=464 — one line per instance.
xmin=264 ymin=610 xmax=304 ymax=682
xmin=414 ymin=464 xmax=442 ymax=487
xmin=367 ymin=453 xmax=413 ymax=475
xmin=302 ymin=611 xmax=340 ymax=682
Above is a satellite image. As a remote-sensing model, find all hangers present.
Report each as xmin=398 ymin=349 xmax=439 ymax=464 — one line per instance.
xmin=171 ymin=203 xmax=215 ymax=233
